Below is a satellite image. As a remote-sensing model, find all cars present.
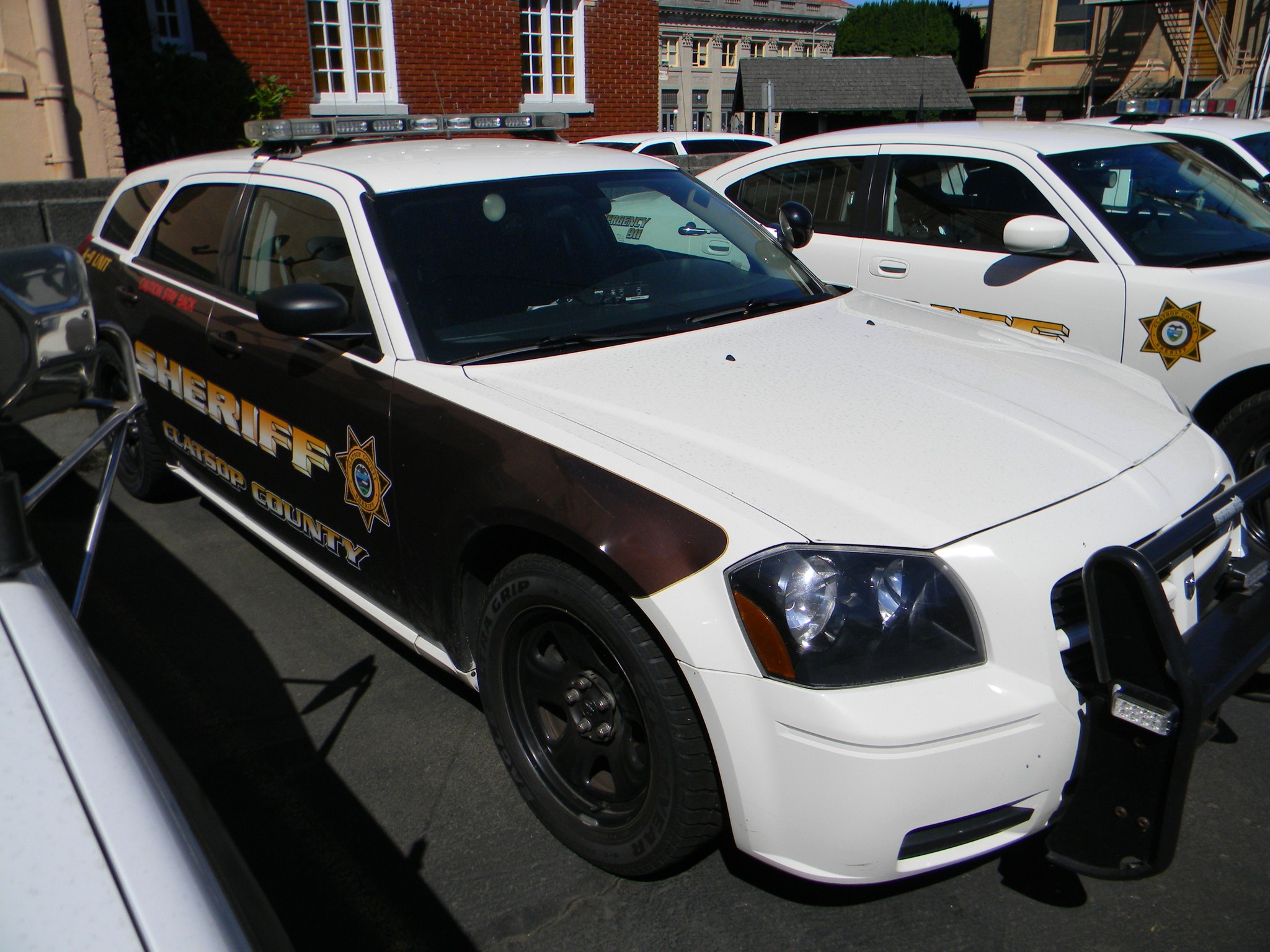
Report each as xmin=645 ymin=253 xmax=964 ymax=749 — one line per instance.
xmin=577 ymin=130 xmax=778 ymax=156
xmin=890 ymin=112 xmax=1270 ymax=236
xmin=605 ymin=114 xmax=1270 ymax=525
xmin=74 ymin=110 xmax=1270 ymax=889
xmin=2 ymin=238 xmax=297 ymax=952
